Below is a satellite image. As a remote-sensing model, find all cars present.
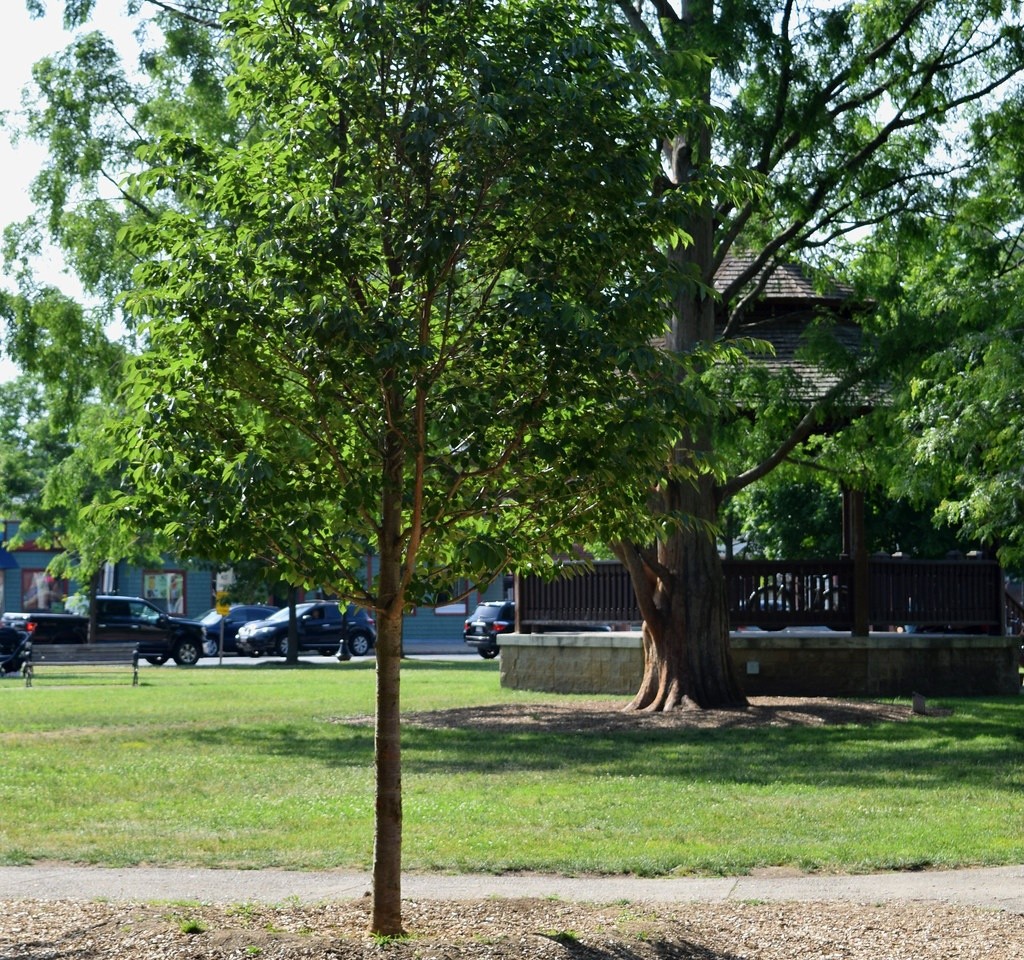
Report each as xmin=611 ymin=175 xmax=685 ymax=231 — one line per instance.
xmin=193 ymin=605 xmax=280 ymax=657
xmin=0 ymin=620 xmax=37 ymax=677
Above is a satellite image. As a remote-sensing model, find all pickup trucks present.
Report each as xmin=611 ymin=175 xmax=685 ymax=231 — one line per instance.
xmin=3 ymin=595 xmax=207 ymax=665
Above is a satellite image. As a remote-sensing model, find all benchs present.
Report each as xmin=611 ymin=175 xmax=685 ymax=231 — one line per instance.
xmin=24 ymin=641 xmax=140 ymax=687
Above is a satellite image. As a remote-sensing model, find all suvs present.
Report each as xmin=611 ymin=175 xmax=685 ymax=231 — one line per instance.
xmin=464 ymin=600 xmax=610 ymax=659
xmin=234 ymin=599 xmax=376 ymax=658
